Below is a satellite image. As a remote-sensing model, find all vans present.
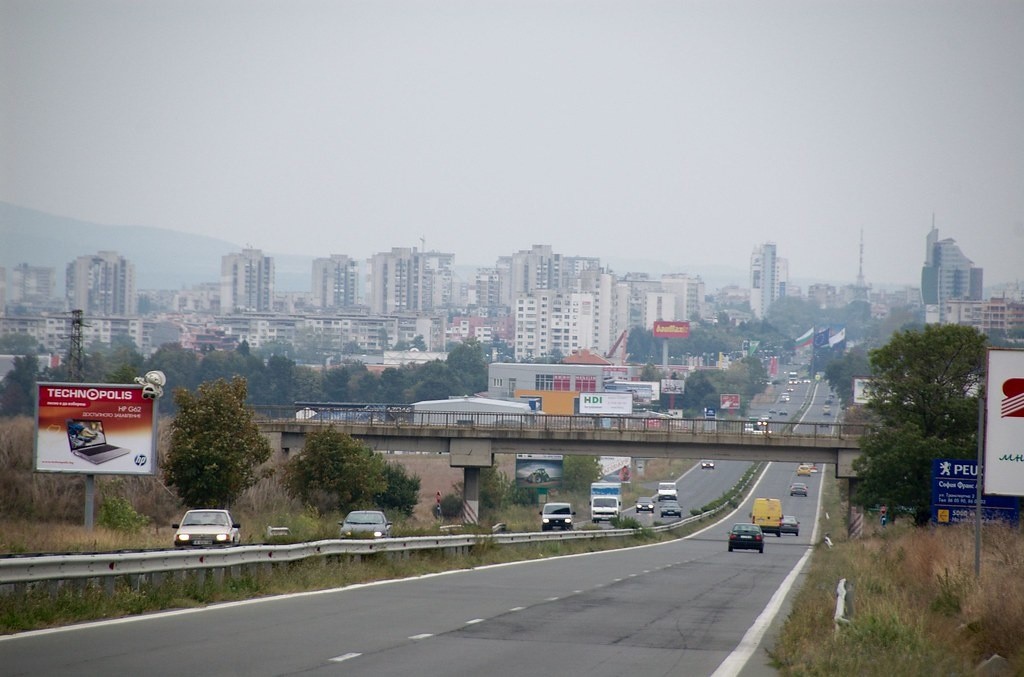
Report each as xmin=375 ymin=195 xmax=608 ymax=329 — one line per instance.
xmin=750 ymin=496 xmax=783 ymax=537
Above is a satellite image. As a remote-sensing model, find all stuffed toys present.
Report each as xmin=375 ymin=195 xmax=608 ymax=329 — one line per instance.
xmin=134 ymin=370 xmax=166 ymax=399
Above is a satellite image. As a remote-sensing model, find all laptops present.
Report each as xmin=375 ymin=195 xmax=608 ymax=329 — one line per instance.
xmin=66 ymin=419 xmax=131 ymax=464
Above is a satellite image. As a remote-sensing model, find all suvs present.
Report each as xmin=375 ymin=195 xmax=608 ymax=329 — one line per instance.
xmin=539 ymin=501 xmax=575 ymax=530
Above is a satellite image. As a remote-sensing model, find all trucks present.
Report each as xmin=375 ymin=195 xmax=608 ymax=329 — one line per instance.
xmin=587 ymin=482 xmax=623 ymax=522
xmin=656 ymin=481 xmax=679 ymax=502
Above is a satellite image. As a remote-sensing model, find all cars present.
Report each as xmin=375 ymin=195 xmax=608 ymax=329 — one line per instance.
xmin=170 ymin=509 xmax=242 ymax=549
xmin=781 ymin=515 xmax=800 ymax=537
xmin=726 ymin=522 xmax=764 ymax=554
xmin=789 ymin=482 xmax=809 ymax=496
xmin=634 ymin=496 xmax=655 ymax=513
xmin=337 ymin=510 xmax=394 ymax=539
xmin=798 ymin=465 xmax=812 ymax=477
xmin=659 ymin=499 xmax=683 ymax=518
xmin=700 ymin=459 xmax=716 ymax=470
xmin=743 ymin=372 xmax=836 ymax=435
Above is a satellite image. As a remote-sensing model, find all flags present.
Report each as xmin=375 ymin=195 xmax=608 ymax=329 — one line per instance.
xmin=828 ymin=327 xmax=846 ymax=348
xmin=794 ymin=326 xmax=813 ymax=350
xmin=814 ymin=329 xmax=829 ymax=348
